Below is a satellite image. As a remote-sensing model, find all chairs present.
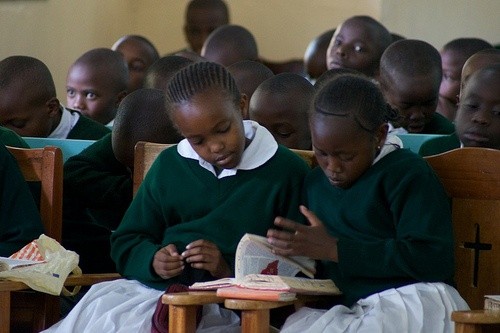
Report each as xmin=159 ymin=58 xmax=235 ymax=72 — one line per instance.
xmin=0 ymin=140 xmax=500 ymax=333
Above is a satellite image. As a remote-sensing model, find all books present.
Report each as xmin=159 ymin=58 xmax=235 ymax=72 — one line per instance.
xmin=0 ymin=257 xmax=47 ymax=272
xmin=189 ymin=233 xmax=342 ymax=295
xmin=216 ymin=287 xmax=299 ymax=302
xmin=483 ymin=295 xmax=500 ymax=311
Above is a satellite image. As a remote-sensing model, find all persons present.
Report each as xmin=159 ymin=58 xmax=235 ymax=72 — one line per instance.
xmin=266 ymin=72 xmax=471 ymax=333
xmin=1 ymin=56 xmax=112 ymax=210
xmin=35 ymin=62 xmax=312 ymax=333
xmin=65 ymin=48 xmax=130 ymax=131
xmin=435 ymin=38 xmax=500 ymax=123
xmin=184 ymin=0 xmax=229 ymax=53
xmin=59 ymin=87 xmax=184 ymax=320
xmin=274 ymin=15 xmax=406 ymax=77
xmin=380 ymin=40 xmax=456 ymax=134
xmin=250 ymin=72 xmax=315 ymax=150
xmin=111 ymin=25 xmax=275 ymax=119
xmin=418 ymin=65 xmax=499 ymax=287
xmin=0 ymin=141 xmax=42 ymax=257
